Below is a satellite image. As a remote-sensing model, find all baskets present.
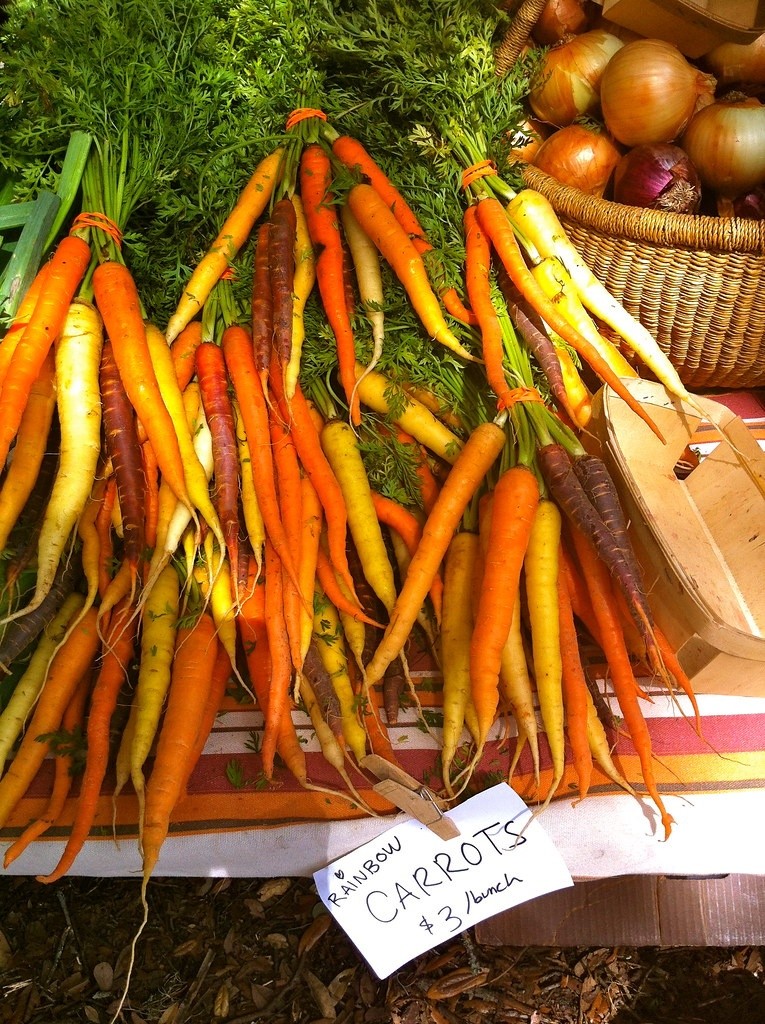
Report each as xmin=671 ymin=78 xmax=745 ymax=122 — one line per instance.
xmin=493 ymin=0 xmax=765 ymax=391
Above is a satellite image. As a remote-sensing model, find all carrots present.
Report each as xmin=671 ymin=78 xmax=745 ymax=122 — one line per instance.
xmin=0 ymin=137 xmax=753 ymax=1024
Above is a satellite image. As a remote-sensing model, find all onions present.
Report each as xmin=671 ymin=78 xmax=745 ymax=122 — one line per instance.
xmin=499 ymin=0 xmax=765 ymax=224
